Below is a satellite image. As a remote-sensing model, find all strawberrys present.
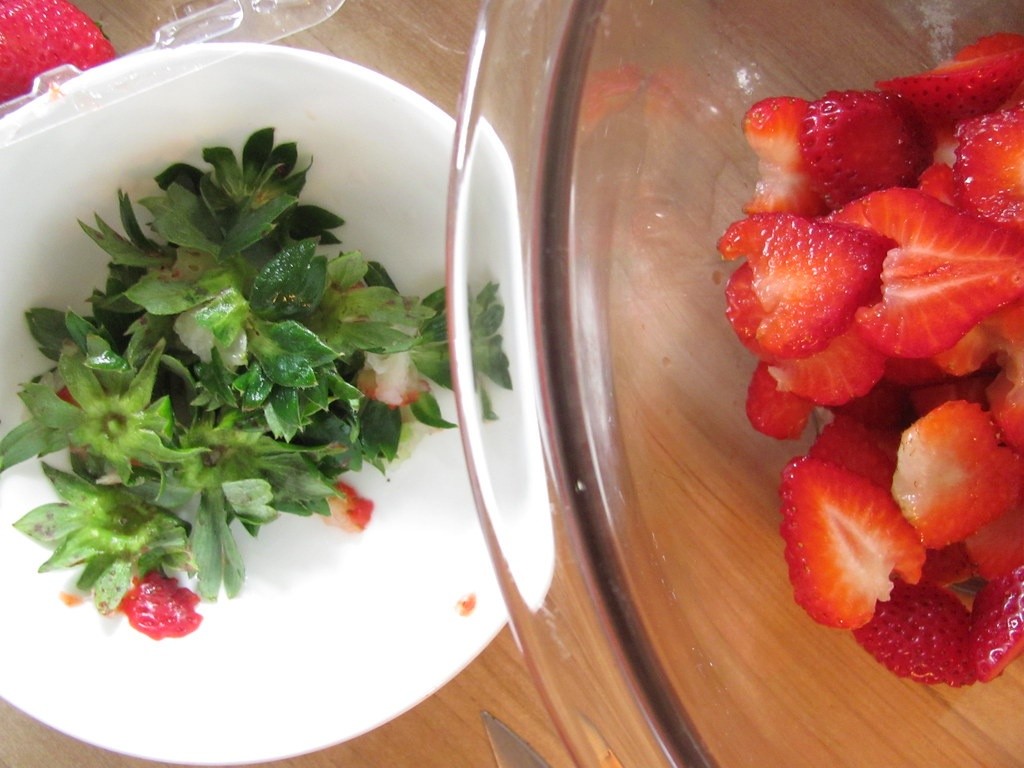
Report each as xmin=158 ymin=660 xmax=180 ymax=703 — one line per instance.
xmin=718 ymin=31 xmax=1024 ymax=688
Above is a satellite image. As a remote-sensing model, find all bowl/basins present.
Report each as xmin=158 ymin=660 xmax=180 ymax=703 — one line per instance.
xmin=446 ymin=2 xmax=1024 ymax=762
xmin=0 ymin=39 xmax=563 ymax=763
